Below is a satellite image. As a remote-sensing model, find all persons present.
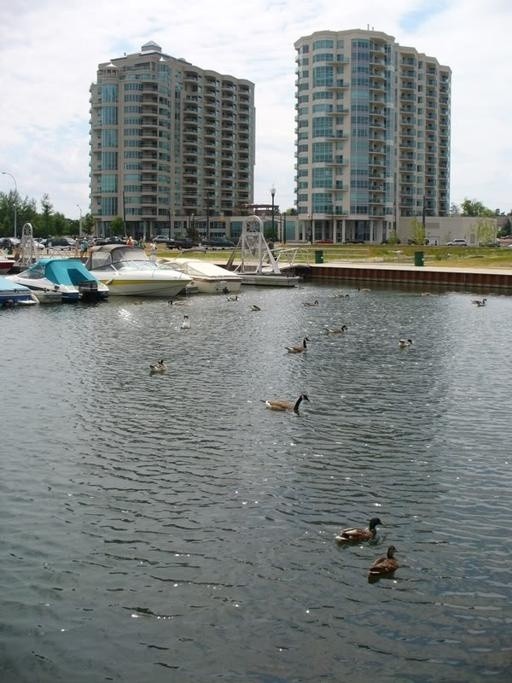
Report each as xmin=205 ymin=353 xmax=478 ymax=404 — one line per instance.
xmin=80 ymin=241 xmax=85 ymax=260
xmin=39 ymin=236 xmax=48 ymax=250
xmin=83 ymin=238 xmax=93 ymax=256
xmin=46 ymin=234 xmax=53 ymax=247
xmin=0 ymin=246 xmax=5 ymax=256
xmin=150 ymin=247 xmax=157 ymax=260
xmin=126 ymin=236 xmax=134 ymax=249
xmin=136 ymin=239 xmax=146 ymax=250
xmin=11 ymin=245 xmax=22 ymax=261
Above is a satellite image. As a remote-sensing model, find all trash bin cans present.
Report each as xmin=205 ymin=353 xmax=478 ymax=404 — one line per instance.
xmin=414 ymin=251 xmax=424 ymax=266
xmin=315 ymin=250 xmax=323 ymax=264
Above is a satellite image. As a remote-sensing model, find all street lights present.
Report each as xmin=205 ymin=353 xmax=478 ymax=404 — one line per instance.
xmin=75 ymin=203 xmax=84 ymax=236
xmin=269 ymin=183 xmax=277 ymax=243
xmin=1 ymin=169 xmax=19 ymax=237
xmin=201 ymin=236 xmax=235 ymax=247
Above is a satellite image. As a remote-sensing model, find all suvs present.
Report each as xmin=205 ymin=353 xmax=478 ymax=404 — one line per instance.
xmin=447 ymin=237 xmax=469 ymax=246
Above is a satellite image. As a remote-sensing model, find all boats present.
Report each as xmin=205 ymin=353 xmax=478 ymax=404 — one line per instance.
xmin=0 ymin=222 xmax=244 ymax=306
xmin=231 ymin=212 xmax=301 ymax=286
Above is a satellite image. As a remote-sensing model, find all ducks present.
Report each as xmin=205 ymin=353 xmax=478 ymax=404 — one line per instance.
xmin=368 ymin=545 xmax=400 ymax=575
xmin=334 ymin=517 xmax=384 ymax=542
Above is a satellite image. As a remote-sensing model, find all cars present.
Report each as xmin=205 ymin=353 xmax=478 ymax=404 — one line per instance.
xmin=342 ymin=239 xmax=365 ymax=245
xmin=409 ymin=238 xmax=429 ymax=244
xmin=478 ymin=239 xmax=501 ymax=247
xmin=380 ymin=238 xmax=402 ymax=245
xmin=166 ymin=237 xmax=193 ymax=250
xmin=315 ymin=238 xmax=334 ymax=243
xmin=150 ymin=233 xmax=174 ymax=243
xmin=0 ymin=234 xmax=130 ymax=250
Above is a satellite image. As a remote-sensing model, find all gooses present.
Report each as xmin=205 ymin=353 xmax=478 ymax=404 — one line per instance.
xmin=284 ymin=337 xmax=312 ymax=353
xmin=259 ymin=392 xmax=311 ymax=419
xmin=168 ymin=300 xmax=191 ymax=329
xmin=150 ymin=358 xmax=168 ymax=372
xmin=302 ymin=286 xmax=371 ymax=307
xmin=472 ymin=299 xmax=487 ymax=306
xmin=323 ymin=324 xmax=347 ymax=333
xmin=227 ymin=294 xmax=261 ymax=312
xmin=400 ymin=339 xmax=413 ymax=347
